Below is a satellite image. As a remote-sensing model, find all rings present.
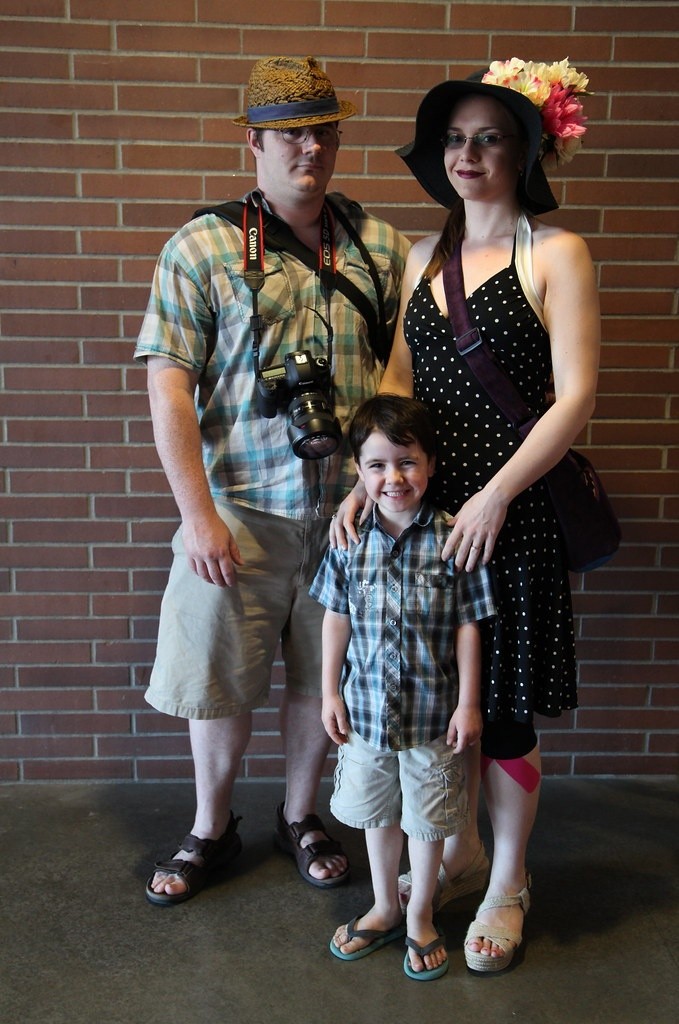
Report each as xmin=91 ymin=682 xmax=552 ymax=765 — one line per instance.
xmin=332 ymin=515 xmax=337 ymax=518
xmin=472 ymin=547 xmax=481 ymax=551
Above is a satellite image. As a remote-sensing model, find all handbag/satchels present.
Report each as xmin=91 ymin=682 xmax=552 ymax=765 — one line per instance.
xmin=552 ymin=448 xmax=622 ymax=574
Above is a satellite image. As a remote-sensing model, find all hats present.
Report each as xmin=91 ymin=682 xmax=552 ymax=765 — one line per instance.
xmin=232 ymin=58 xmax=358 ymax=130
xmin=394 ymin=56 xmax=595 ymax=216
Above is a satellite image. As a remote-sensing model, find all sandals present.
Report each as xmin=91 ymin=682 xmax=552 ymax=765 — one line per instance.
xmin=145 ymin=810 xmax=244 ymax=907
xmin=276 ymin=801 xmax=353 ymax=890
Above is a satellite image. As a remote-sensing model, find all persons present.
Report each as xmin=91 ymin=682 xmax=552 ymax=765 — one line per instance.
xmin=308 ymin=393 xmax=498 ymax=979
xmin=328 ymin=54 xmax=601 ymax=975
xmin=133 ymin=56 xmax=412 ymax=907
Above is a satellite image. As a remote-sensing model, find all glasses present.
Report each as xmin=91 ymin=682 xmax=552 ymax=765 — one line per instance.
xmin=281 ymin=128 xmax=343 ymax=145
xmin=438 ymin=132 xmax=505 ymax=148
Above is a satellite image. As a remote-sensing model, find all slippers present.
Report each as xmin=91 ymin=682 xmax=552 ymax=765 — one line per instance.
xmin=403 ymin=918 xmax=450 ymax=981
xmin=329 ymin=912 xmax=410 ymax=961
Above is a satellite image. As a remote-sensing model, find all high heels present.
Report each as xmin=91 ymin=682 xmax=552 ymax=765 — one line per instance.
xmin=463 ymin=870 xmax=534 ymax=971
xmin=397 ymin=841 xmax=489 ymax=916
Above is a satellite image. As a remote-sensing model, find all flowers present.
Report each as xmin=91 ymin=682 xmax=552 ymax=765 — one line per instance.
xmin=480 ymin=56 xmax=595 ymax=166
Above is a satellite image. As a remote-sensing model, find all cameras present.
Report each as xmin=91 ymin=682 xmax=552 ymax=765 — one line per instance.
xmin=257 ymin=350 xmax=341 ymax=459
